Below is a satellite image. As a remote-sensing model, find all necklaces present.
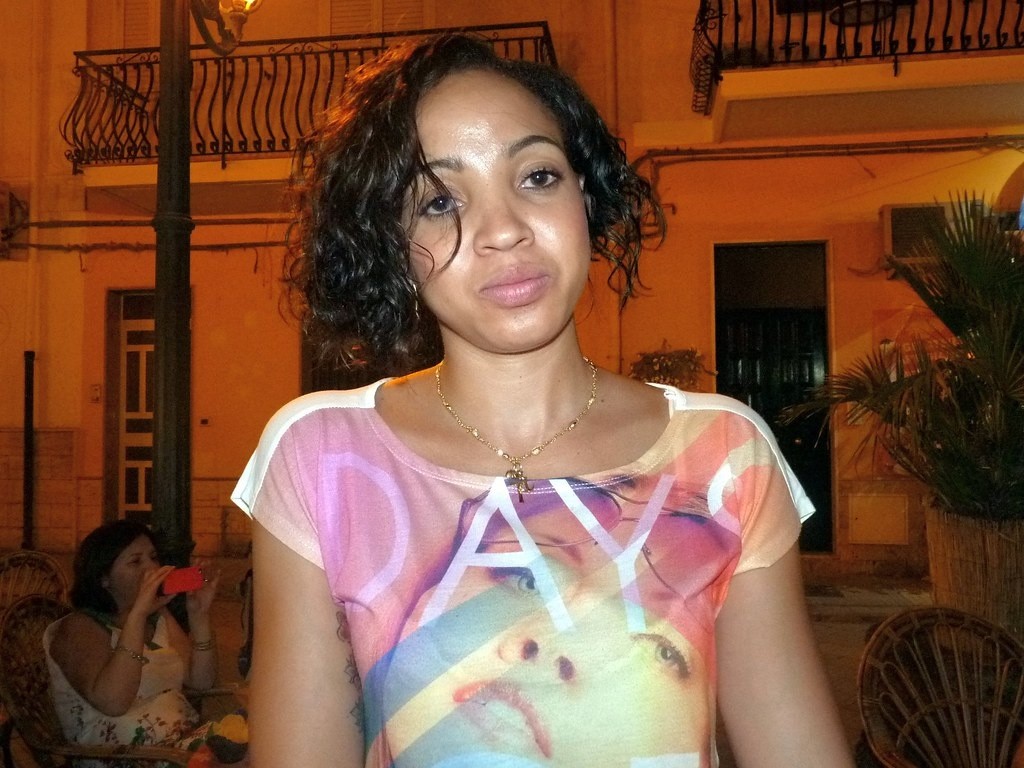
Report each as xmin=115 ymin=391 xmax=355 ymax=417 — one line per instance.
xmin=436 ymin=354 xmax=598 ymax=481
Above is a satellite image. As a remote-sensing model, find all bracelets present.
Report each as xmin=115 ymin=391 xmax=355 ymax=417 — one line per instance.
xmin=113 ymin=647 xmax=149 ymax=665
xmin=191 ymin=630 xmax=216 ymax=651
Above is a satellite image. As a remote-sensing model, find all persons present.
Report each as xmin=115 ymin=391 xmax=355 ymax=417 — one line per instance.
xmin=44 ymin=520 xmax=222 ymax=768
xmin=229 ymin=28 xmax=855 ymax=768
xmin=384 ymin=477 xmax=711 ymax=768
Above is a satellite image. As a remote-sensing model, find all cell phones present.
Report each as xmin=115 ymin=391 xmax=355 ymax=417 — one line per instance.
xmin=162 ymin=567 xmax=204 ymax=595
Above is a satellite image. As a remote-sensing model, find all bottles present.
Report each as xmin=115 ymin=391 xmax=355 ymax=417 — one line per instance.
xmin=189 ymin=709 xmax=249 ymax=767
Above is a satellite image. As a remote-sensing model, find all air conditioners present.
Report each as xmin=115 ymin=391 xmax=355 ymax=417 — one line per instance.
xmin=878 ymin=197 xmax=987 ymax=264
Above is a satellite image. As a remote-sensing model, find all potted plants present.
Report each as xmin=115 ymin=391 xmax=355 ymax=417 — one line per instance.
xmin=769 ymin=186 xmax=1024 ymax=648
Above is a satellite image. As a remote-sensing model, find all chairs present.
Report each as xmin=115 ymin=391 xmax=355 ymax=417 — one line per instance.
xmin=858 ymin=603 xmax=1024 ymax=768
xmin=0 ymin=592 xmax=196 ymax=768
xmin=0 ymin=548 xmax=70 ymax=768
